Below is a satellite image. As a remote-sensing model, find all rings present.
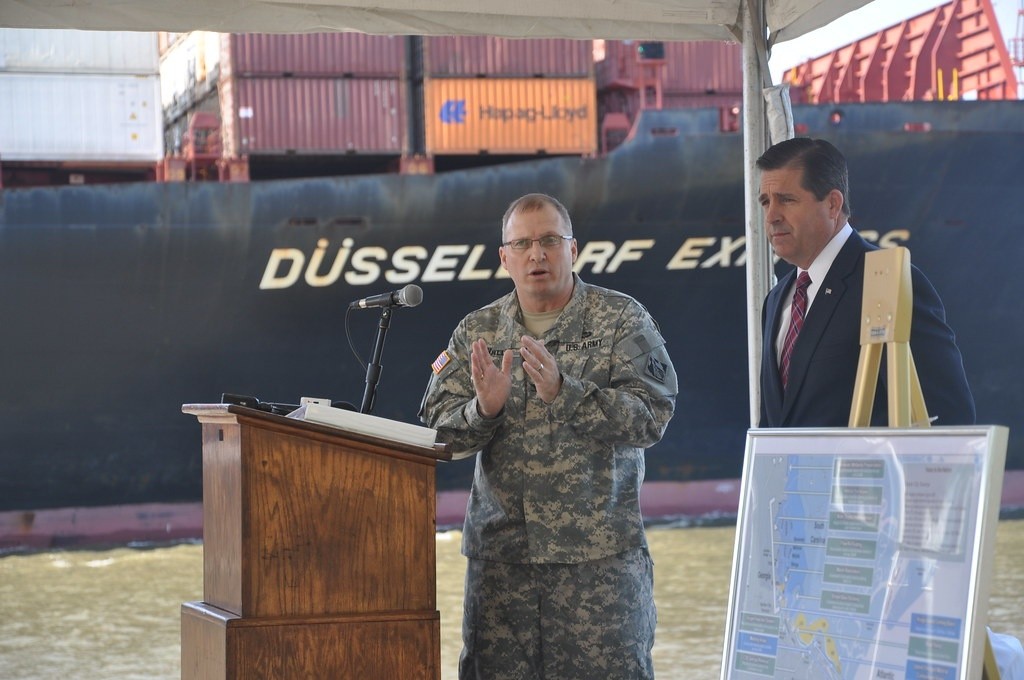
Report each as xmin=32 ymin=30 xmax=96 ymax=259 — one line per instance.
xmin=537 ymin=364 xmax=544 ymax=372
xmin=481 ymin=374 xmax=484 ymax=379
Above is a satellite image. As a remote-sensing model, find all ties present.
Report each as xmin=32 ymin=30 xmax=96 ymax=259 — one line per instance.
xmin=779 ymin=271 xmax=812 ymax=392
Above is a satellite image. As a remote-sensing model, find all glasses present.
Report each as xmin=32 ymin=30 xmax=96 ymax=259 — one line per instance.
xmin=503 ymin=235 xmax=573 ymax=249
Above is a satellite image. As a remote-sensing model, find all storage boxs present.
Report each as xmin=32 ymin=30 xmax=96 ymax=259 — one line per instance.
xmin=155 ymin=31 xmax=741 ymax=163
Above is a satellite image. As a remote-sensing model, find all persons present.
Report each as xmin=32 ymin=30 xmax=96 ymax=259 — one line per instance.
xmin=753 ymin=135 xmax=980 ymax=428
xmin=416 ymin=194 xmax=677 ymax=680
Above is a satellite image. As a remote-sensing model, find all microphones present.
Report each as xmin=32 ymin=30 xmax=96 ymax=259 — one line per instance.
xmin=350 ymin=284 xmax=424 ymax=310
xmin=221 ymin=392 xmax=358 ymax=417
